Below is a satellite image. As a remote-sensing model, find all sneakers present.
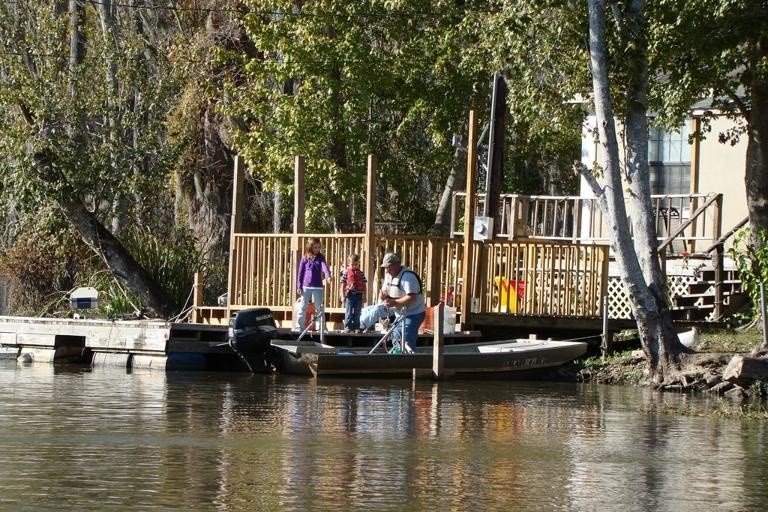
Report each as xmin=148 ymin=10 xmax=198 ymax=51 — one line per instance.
xmin=295 ymin=327 xmax=362 ymax=334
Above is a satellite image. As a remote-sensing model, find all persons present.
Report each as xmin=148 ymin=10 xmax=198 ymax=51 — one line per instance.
xmin=379 ymin=252 xmax=426 ymax=353
xmin=295 ymin=237 xmax=334 ymax=332
xmin=340 ymin=253 xmax=367 ymax=334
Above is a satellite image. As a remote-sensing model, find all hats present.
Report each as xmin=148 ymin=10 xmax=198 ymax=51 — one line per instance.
xmin=378 ymin=253 xmax=400 ymax=268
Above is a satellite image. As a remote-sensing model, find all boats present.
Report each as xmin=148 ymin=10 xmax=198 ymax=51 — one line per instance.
xmin=220 ymin=302 xmax=592 ymax=381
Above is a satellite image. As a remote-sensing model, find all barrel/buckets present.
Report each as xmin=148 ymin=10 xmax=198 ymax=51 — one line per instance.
xmin=418 ymin=308 xmax=434 ymax=335
xmin=291 ymin=297 xmax=315 ymax=330
xmin=434 ymin=307 xmax=457 ymax=335
